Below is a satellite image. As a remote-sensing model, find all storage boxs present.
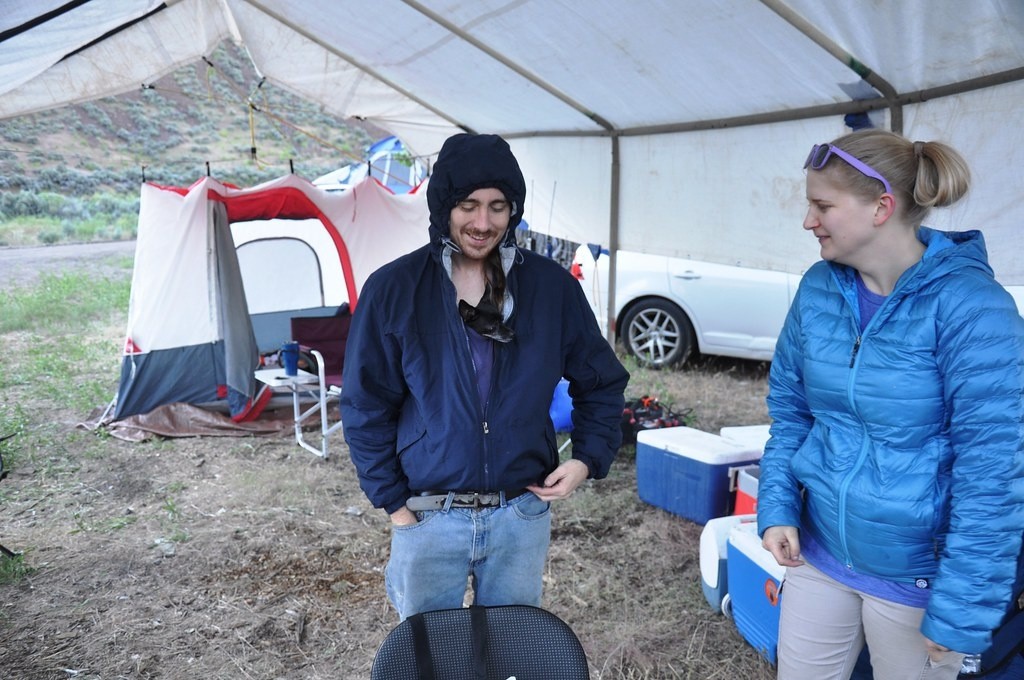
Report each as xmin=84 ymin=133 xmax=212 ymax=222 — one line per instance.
xmin=719 ymin=426 xmax=771 ymax=450
xmin=636 ymin=425 xmax=763 ymax=526
xmin=698 ymin=514 xmax=759 ymax=616
xmin=735 ymin=466 xmax=762 ymax=516
xmin=726 ymin=523 xmax=780 ymax=667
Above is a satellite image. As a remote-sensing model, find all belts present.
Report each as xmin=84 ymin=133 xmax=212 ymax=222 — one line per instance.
xmin=406 ymin=488 xmax=529 ymax=511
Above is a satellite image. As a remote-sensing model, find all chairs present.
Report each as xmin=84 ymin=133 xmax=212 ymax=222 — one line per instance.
xmin=371 ymin=604 xmax=589 ymax=680
xmin=291 ymin=316 xmax=351 ymax=463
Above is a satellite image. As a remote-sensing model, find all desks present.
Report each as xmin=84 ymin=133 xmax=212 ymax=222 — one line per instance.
xmin=254 ymin=368 xmax=318 ymax=388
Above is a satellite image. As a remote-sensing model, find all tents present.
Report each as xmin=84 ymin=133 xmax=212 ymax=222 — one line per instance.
xmin=115 ymin=173 xmax=430 ymax=423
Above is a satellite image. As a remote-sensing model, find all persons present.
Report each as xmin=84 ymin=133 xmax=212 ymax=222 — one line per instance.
xmin=339 ymin=133 xmax=630 ymax=623
xmin=756 ymin=128 xmax=1024 ymax=680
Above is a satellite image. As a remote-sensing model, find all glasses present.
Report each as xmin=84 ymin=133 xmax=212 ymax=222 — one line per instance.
xmin=803 ymin=144 xmax=892 ymax=194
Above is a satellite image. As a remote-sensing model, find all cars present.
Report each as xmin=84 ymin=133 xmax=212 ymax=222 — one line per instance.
xmin=573 ymin=244 xmax=801 ymax=371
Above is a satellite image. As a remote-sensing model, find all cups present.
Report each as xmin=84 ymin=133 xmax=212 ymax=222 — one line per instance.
xmin=280 ymin=341 xmax=300 ymax=376
